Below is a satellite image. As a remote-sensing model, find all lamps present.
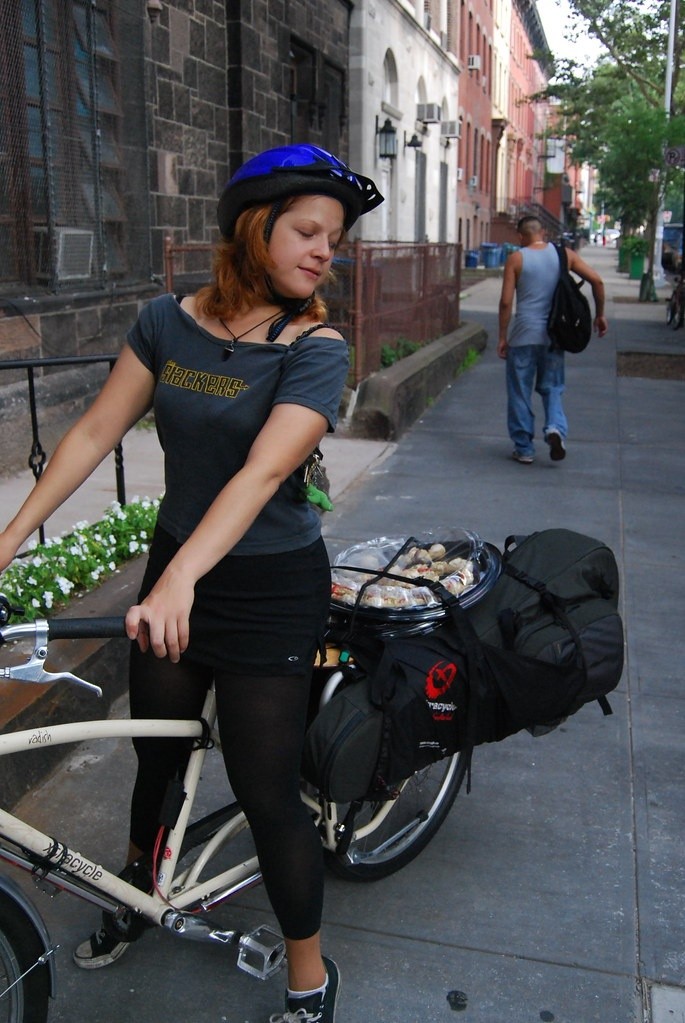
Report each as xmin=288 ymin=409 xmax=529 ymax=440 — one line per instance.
xmin=376 ymin=114 xmax=396 ymax=158
xmin=403 ymin=130 xmax=422 ymax=147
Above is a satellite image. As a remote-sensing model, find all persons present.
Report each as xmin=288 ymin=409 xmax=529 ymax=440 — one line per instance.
xmin=497 ymin=217 xmax=608 ymax=464
xmin=1 ymin=148 xmax=385 ymax=1023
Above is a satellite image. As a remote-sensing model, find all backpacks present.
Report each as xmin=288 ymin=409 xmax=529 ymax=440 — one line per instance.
xmin=547 ymin=243 xmax=593 ymax=353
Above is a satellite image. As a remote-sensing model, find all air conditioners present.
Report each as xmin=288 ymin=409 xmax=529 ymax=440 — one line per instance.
xmin=468 ymin=55 xmax=481 ymax=70
xmin=472 ymin=176 xmax=478 ymax=186
xmin=33 ymin=226 xmax=94 ymax=282
xmin=417 ymin=104 xmax=441 ymax=124
xmin=458 ymin=168 xmax=463 ymax=180
xmin=441 ymin=120 xmax=462 ymax=139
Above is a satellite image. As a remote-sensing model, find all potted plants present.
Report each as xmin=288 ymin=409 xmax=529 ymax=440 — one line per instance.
xmin=618 ymin=235 xmax=651 ymax=279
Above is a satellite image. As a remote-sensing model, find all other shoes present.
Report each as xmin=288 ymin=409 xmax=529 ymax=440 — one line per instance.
xmin=544 ymin=429 xmax=566 ymax=461
xmin=511 ymin=448 xmax=534 ymax=464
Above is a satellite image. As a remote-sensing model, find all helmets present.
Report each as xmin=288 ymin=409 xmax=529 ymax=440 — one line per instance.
xmin=216 ymin=143 xmax=384 ymax=247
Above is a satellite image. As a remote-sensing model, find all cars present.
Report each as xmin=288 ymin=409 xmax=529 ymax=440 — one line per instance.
xmin=601 ymin=229 xmax=621 ymax=240
xmin=671 ymin=234 xmax=683 ymax=271
xmin=663 ymin=222 xmax=684 ymax=265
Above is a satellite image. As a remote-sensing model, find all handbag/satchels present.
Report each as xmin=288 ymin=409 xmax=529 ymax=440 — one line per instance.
xmin=512 ymin=598 xmax=623 ymax=738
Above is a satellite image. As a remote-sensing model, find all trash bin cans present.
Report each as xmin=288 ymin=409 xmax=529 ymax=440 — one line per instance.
xmin=466 ymin=243 xmax=520 ymax=269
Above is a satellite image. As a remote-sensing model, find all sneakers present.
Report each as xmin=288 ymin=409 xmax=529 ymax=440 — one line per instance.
xmin=72 ymin=925 xmax=130 ymax=970
xmin=269 ymin=954 xmax=341 ymax=1023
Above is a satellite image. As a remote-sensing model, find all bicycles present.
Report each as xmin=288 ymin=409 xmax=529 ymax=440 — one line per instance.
xmin=665 ymin=271 xmax=685 ymax=330
xmin=0 ymin=591 xmax=475 ymax=1022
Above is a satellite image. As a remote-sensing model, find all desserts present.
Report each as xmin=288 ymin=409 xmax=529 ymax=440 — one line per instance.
xmin=331 ymin=543 xmax=474 ymax=606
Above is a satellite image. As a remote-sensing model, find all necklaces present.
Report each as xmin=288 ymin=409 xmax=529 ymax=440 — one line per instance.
xmin=215 ymin=311 xmax=282 ymax=352
